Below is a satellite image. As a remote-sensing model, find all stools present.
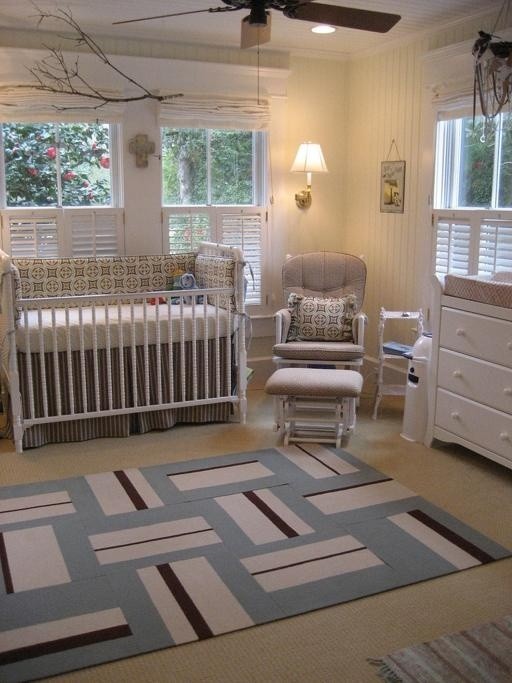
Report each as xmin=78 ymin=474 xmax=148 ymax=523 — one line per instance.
xmin=264 ymin=367 xmax=364 ymax=450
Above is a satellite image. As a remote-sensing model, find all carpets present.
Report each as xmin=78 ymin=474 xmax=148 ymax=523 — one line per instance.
xmin=1 ymin=442 xmax=512 ymax=683
xmin=366 ymin=615 xmax=512 ymax=683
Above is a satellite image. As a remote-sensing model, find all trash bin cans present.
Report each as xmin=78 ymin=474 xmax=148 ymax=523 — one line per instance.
xmin=399 ymin=333 xmax=433 ymax=442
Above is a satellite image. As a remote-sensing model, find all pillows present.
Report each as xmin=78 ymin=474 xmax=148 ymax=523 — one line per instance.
xmin=286 ymin=293 xmax=358 ymax=342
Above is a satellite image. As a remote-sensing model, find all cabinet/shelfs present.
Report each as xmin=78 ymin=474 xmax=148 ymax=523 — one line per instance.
xmin=423 ymin=270 xmax=512 ymax=471
xmin=371 ymin=306 xmax=423 ymax=420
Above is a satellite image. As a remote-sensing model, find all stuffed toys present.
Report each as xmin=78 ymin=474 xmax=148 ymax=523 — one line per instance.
xmin=169 ymin=272 xmax=206 ymax=305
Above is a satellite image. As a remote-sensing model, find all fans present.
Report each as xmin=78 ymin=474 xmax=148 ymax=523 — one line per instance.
xmin=111 ymin=0 xmax=401 ymax=50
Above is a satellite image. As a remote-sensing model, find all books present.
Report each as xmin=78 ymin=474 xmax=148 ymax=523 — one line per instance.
xmin=383 ymin=339 xmax=413 ymax=355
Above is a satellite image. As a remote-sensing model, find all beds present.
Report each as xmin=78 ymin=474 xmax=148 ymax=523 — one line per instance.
xmin=0 ymin=240 xmax=247 ymax=454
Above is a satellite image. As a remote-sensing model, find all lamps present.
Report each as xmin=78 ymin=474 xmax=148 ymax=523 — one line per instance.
xmin=290 ymin=142 xmax=329 ymax=209
xmin=248 ymin=2 xmax=267 ymax=107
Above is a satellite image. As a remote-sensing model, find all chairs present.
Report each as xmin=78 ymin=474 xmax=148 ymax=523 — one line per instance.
xmin=271 ymin=251 xmax=368 ymax=436
